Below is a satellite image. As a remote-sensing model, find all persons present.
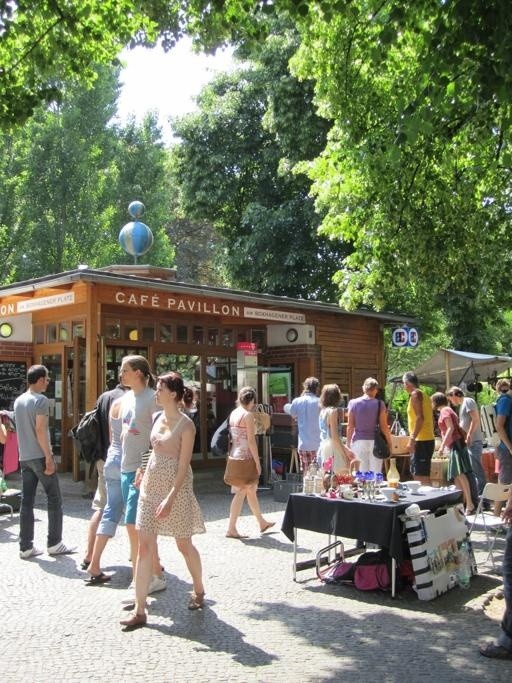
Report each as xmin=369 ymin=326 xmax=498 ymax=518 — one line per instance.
xmin=119 ymin=373 xmax=207 ymax=627
xmin=445 ymin=386 xmax=492 ymax=512
xmin=223 ymin=386 xmax=276 ymax=538
xmin=475 ymin=487 xmax=512 ymax=660
xmin=402 ymin=371 xmax=436 ymax=485
xmin=491 ymin=378 xmax=512 ymax=517
xmin=289 ymin=377 xmax=321 ymax=474
xmin=347 ymin=377 xmax=393 ymax=474
xmin=84 ymin=386 xmax=132 ymax=583
xmin=80 ymin=373 xmax=130 ymax=570
xmin=316 ymin=384 xmax=354 ymax=490
xmin=429 ymin=392 xmax=476 ymax=516
xmin=13 ymin=364 xmax=78 ymax=558
xmin=1 ymin=409 xmax=9 ymax=496
xmin=118 ymin=355 xmax=167 ymax=604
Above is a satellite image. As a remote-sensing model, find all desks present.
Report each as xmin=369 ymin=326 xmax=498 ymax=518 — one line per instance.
xmin=281 ymin=487 xmax=464 ymax=600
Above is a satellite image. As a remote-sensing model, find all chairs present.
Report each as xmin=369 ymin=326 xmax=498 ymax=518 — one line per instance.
xmin=466 ymin=482 xmax=512 ymax=569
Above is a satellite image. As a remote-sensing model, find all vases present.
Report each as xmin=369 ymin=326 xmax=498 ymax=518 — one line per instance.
xmin=387 ymin=458 xmax=401 ymax=486
xmin=323 ymin=472 xmax=340 ymax=492
xmin=363 ymin=480 xmax=375 ymax=502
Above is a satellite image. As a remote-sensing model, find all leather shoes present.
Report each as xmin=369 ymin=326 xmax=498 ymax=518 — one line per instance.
xmin=119 ymin=613 xmax=147 ymax=626
xmin=188 ymin=590 xmax=206 ymax=608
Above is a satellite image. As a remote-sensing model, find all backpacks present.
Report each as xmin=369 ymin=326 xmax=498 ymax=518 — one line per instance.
xmin=68 ymin=402 xmax=101 ymax=480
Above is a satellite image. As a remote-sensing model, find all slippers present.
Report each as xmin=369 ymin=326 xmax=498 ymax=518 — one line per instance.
xmin=226 ymin=534 xmax=250 ymax=539
xmin=465 ymin=505 xmax=475 ymax=516
xmin=80 ymin=558 xmax=92 ymax=567
xmin=260 ymin=522 xmax=275 ymax=532
xmin=83 ymin=570 xmax=111 ymax=584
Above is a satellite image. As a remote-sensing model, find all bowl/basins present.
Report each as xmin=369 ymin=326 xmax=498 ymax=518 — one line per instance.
xmin=381 ymin=488 xmax=397 ymax=501
xmin=406 ymin=481 xmax=421 ymax=494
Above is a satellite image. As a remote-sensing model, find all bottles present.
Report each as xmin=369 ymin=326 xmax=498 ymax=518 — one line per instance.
xmin=386 ymin=458 xmax=400 ymax=500
xmin=457 ymin=544 xmax=471 ymax=591
xmin=314 ymin=471 xmax=321 ymax=492
xmin=399 ymin=428 xmax=404 ymax=436
xmin=303 ymin=471 xmax=314 ymax=495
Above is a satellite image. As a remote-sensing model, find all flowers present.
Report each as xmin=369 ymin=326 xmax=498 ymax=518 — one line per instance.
xmin=356 ymin=470 xmax=384 ymax=484
xmin=323 ymin=456 xmax=334 ymax=473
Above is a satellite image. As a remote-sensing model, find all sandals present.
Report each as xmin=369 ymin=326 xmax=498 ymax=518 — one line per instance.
xmin=474 ymin=639 xmax=510 ymax=661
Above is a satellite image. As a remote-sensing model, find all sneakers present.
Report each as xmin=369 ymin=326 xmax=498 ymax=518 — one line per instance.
xmin=48 ymin=540 xmax=78 ymax=555
xmin=25 ymin=545 xmax=42 ymax=558
xmin=146 ymin=575 xmax=167 ymax=593
